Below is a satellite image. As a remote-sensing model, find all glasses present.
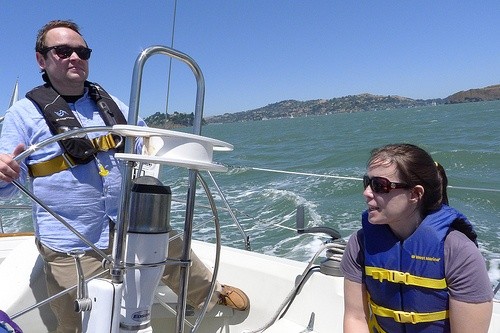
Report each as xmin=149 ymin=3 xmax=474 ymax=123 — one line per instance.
xmin=41 ymin=44 xmax=92 ymax=60
xmin=362 ymin=175 xmax=412 ymax=194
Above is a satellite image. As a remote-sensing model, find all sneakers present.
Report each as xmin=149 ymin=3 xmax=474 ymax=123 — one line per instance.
xmin=216 ymin=284 xmax=250 ymax=311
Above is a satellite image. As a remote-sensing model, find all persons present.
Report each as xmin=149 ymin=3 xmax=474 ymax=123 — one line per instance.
xmin=0 ymin=19 xmax=251 ymax=333
xmin=339 ymin=143 xmax=494 ymax=333
